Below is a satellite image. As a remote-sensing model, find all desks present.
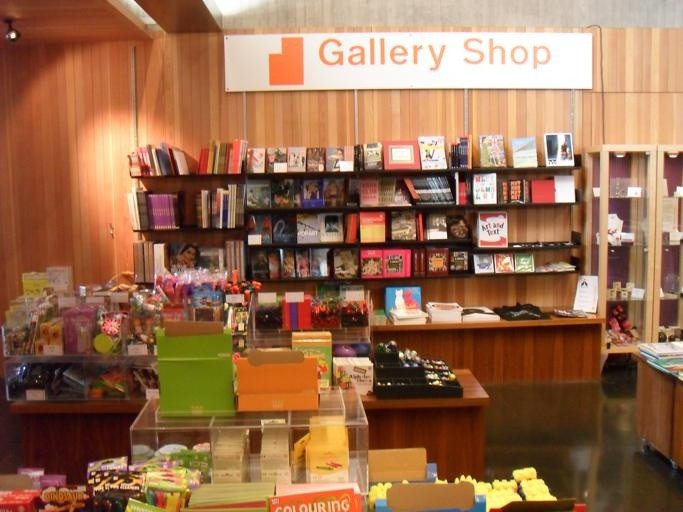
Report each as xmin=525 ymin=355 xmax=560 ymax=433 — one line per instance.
xmin=8 ymin=369 xmax=490 ymax=484
xmin=630 ymin=348 xmax=683 ymax=472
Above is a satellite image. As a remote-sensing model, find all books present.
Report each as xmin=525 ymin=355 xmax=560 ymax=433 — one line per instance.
xmin=573 ymin=276 xmax=600 ymax=314
xmin=291 ymin=331 xmax=332 ymax=389
xmin=246 ymin=135 xmax=574 ymax=174
xmin=256 ymin=283 xmax=367 ymax=335
xmin=128 ymin=182 xmax=244 ymax=230
xmin=246 ymin=172 xmax=472 ymax=208
xmin=333 ymin=357 xmax=374 ymax=394
xmin=476 ymin=210 xmax=508 ymax=249
xmin=473 ymin=172 xmax=576 ymax=204
xmin=372 ymin=286 xmax=500 ymax=326
xmin=133 ymin=240 xmax=246 ymax=283
xmin=638 ymin=340 xmax=683 ymax=381
xmin=250 ymin=246 xmax=577 ymax=278
xmin=128 ymin=139 xmax=248 ymax=176
xmin=245 ymin=211 xmax=472 ymax=246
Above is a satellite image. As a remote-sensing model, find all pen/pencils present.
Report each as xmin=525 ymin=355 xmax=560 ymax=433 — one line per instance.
xmin=145 ymin=489 xmax=192 ymax=512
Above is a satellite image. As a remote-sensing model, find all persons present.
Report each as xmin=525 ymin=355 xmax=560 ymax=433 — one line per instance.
xmin=325 ymin=215 xmax=339 ymax=232
xmin=179 ymin=244 xmax=199 ymax=270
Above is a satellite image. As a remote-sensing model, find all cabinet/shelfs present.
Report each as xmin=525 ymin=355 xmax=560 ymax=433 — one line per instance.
xmin=369 ymin=305 xmax=602 ymax=456
xmin=651 ymin=143 xmax=682 ymax=345
xmin=582 ymin=144 xmax=658 ymax=373
xmin=129 ymin=143 xmax=583 ymax=289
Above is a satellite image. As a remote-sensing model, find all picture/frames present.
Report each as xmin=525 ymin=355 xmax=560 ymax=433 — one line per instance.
xmin=383 ymin=140 xmax=420 ymax=171
